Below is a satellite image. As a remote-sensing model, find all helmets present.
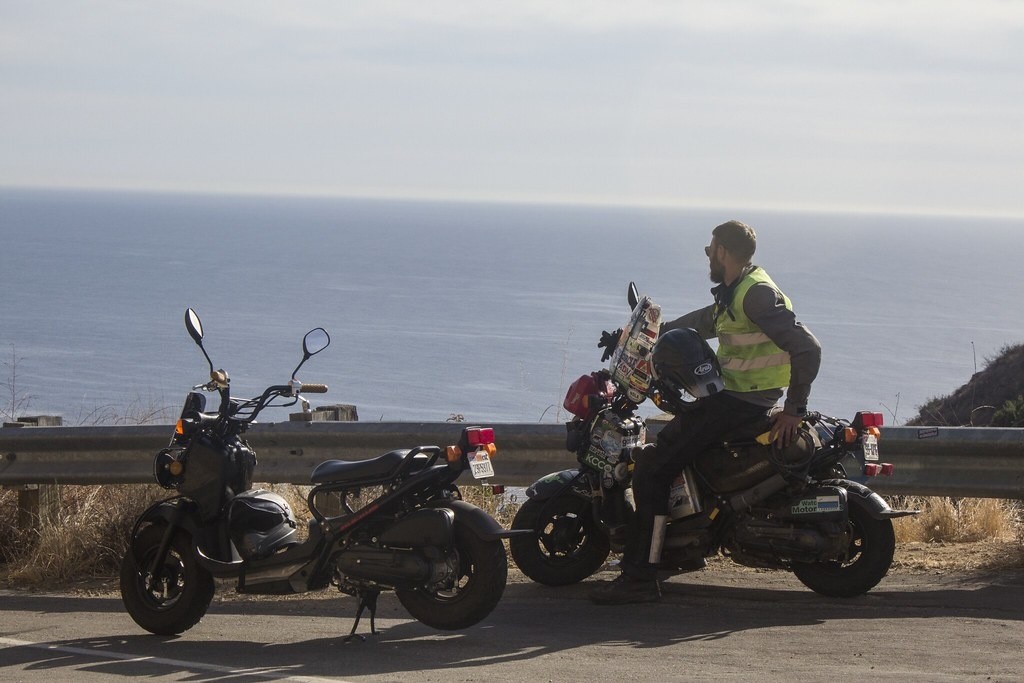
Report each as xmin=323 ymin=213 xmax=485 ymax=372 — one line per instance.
xmin=224 ymin=488 xmax=298 ymax=561
xmin=650 ymin=327 xmax=722 ymax=413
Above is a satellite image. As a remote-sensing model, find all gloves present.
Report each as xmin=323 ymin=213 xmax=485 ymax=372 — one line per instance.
xmin=598 ymin=328 xmax=623 ymax=362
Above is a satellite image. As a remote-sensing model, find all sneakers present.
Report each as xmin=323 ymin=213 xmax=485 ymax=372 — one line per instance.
xmin=590 ymin=569 xmax=661 ymax=605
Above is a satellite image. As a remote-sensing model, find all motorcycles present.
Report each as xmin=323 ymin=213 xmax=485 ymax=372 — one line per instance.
xmin=511 ymin=281 xmax=921 ymax=599
xmin=116 ymin=308 xmax=535 ymax=635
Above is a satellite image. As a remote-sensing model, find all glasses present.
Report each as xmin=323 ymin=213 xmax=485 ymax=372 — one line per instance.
xmin=705 ymin=246 xmax=736 ymax=257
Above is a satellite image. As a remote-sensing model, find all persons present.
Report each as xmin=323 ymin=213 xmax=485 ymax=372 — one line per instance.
xmin=585 ymin=220 xmax=823 ymax=605
